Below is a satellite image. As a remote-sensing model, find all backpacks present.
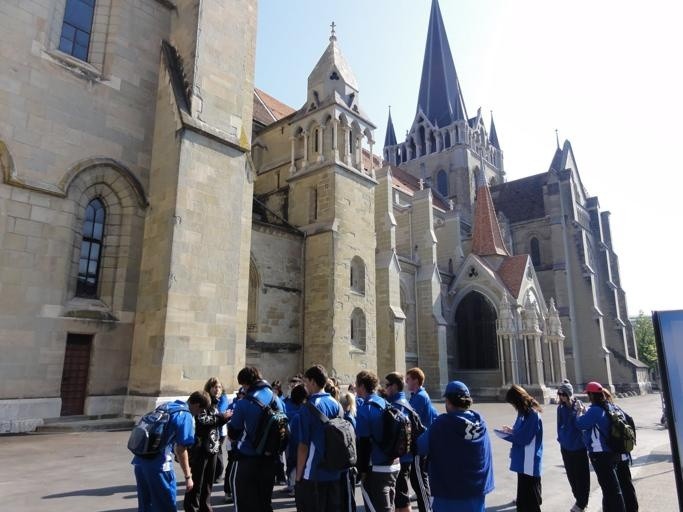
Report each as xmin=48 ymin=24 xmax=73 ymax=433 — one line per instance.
xmin=305 ymin=397 xmax=356 ymax=472
xmin=242 ymin=390 xmax=288 ymax=455
xmin=393 ymin=399 xmax=426 ymax=448
xmin=591 ymin=403 xmax=637 ymax=453
xmin=127 ymin=404 xmax=193 ymax=457
xmin=361 ymin=397 xmax=411 ymax=455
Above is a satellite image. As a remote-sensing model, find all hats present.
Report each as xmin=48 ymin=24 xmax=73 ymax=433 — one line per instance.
xmin=582 ymin=381 xmax=603 ymax=394
xmin=442 ymin=381 xmax=469 ymax=397
xmin=558 ymin=379 xmax=574 ymax=394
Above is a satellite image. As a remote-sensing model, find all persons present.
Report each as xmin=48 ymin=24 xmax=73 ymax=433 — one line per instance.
xmin=223 ymin=366 xmax=285 ymax=512
xmin=352 ymin=368 xmax=435 ymax=512
xmin=279 ymin=364 xmax=360 ymax=512
xmin=129 ymin=377 xmax=230 ymax=512
xmin=555 ymin=378 xmax=589 ymax=511
xmin=502 ymin=383 xmax=544 ymax=511
xmin=416 ymin=381 xmax=495 ymax=511
xmin=601 ymin=386 xmax=637 ymax=511
xmin=575 ymin=382 xmax=626 ymax=510
